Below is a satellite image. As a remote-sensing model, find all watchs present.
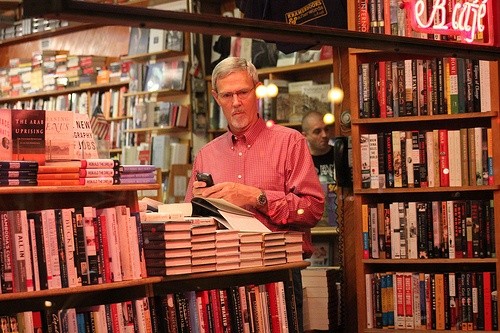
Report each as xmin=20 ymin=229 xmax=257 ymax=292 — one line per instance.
xmin=253 ymin=189 xmax=266 ymax=209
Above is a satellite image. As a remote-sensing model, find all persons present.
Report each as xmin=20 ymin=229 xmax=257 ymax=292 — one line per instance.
xmin=185 ymin=57 xmax=325 ymax=258
xmin=300 ymin=112 xmax=353 ymax=226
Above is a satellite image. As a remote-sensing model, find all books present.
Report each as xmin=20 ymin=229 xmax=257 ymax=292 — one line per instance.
xmin=361 ymin=199 xmax=495 ymax=259
xmin=208 ymin=73 xmax=335 ymax=125
xmin=366 ymin=271 xmax=498 ymax=330
xmin=139 ymin=196 xmax=304 ymax=275
xmin=0 ymin=280 xmax=288 ymax=333
xmin=356 ymin=55 xmax=492 ymax=119
xmin=308 ymin=227 xmax=333 ymax=268
xmin=360 ymin=125 xmax=494 ymax=189
xmin=355 ymin=0 xmax=495 ymax=47
xmin=0 ymin=17 xmax=69 ymax=42
xmin=0 ymin=205 xmax=147 ymax=294
xmin=128 ymin=0 xmax=208 ymax=197
xmin=208 ymin=7 xmax=334 ymax=73
xmin=0 ymin=86 xmax=150 ymax=165
xmin=302 ymin=265 xmax=352 ymax=333
xmin=0 ymin=49 xmax=132 ymax=99
xmin=0 ymin=157 xmax=157 ymax=186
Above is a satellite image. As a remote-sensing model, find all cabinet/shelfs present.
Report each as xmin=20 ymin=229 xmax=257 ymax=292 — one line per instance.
xmin=0 ymin=0 xmax=500 ymax=333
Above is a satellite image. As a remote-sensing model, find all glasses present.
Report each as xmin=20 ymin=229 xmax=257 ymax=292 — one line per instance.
xmin=215 ymin=82 xmax=258 ymax=101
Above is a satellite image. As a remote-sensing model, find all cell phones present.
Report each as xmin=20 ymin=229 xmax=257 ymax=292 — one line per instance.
xmin=196 ymin=173 xmax=214 ymax=187
